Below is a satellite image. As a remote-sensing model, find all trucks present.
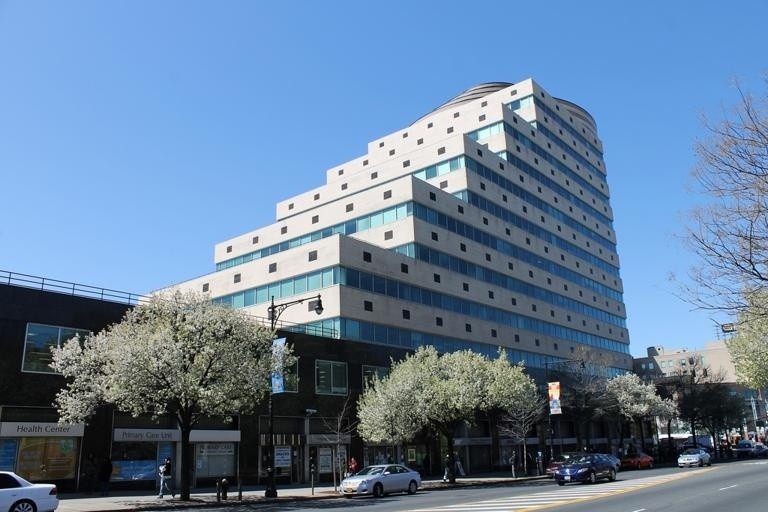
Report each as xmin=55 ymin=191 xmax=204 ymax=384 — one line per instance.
xmin=679 ymin=435 xmax=714 ymax=448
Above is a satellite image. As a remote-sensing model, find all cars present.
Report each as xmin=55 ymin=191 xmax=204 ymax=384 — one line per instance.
xmin=0 ymin=471 xmax=59 ymax=512
xmin=619 ymin=453 xmax=654 ymax=470
xmin=677 ymin=443 xmax=711 ymax=468
xmin=339 ymin=464 xmax=422 ymax=498
xmin=731 ymin=440 xmax=768 ymax=458
xmin=546 ymin=451 xmax=621 ymax=486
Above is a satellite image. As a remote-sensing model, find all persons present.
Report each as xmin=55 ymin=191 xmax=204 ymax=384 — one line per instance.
xmin=508 ymin=454 xmax=518 ymax=478
xmin=720 ymin=432 xmax=767 ymax=451
xmin=157 ymin=457 xmax=176 ymax=500
xmin=535 ymin=450 xmax=545 ymax=476
xmin=347 ymin=456 xmax=357 ymax=477
xmin=441 ymin=454 xmax=450 ymax=482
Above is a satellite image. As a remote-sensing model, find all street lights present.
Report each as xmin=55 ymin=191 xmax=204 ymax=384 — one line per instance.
xmin=264 ymin=294 xmax=323 ymax=498
xmin=543 ymin=355 xmax=585 ymax=462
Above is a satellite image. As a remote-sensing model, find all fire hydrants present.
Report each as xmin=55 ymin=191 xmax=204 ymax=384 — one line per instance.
xmin=221 ymin=479 xmax=229 ymax=499
